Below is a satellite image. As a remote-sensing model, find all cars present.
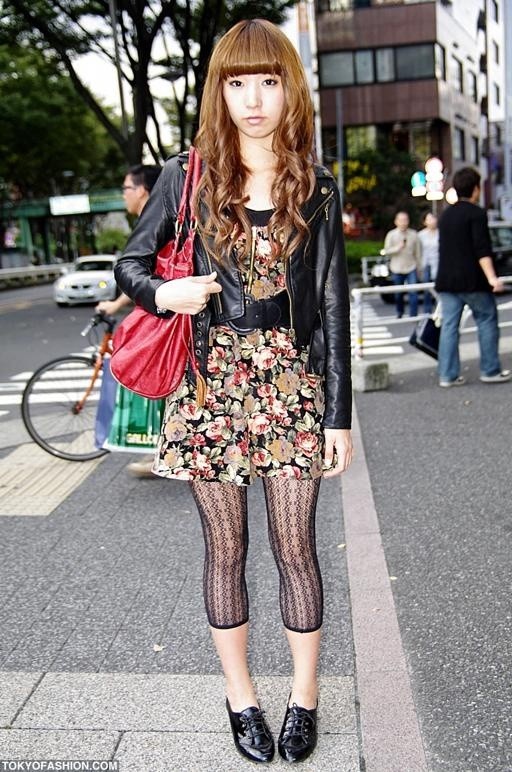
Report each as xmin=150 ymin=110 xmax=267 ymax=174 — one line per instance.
xmin=52 ymin=253 xmax=119 ymax=308
xmin=368 ymin=221 xmax=512 ymax=303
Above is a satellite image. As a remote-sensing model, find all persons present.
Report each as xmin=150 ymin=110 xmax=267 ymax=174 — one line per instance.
xmin=92 ymin=164 xmax=176 ymax=482
xmin=384 ymin=211 xmax=422 ymax=321
xmin=112 ymin=14 xmax=354 ymax=767
xmin=435 ymin=167 xmax=512 ymax=386
xmin=417 ymin=211 xmax=441 ymax=313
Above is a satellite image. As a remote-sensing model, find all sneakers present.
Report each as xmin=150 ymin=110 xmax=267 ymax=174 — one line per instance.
xmin=479 ymin=370 xmax=510 ymax=382
xmin=440 ymin=376 xmax=465 ymax=386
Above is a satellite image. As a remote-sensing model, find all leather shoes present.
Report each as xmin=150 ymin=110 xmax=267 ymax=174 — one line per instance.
xmin=278 ymin=692 xmax=318 ymax=762
xmin=226 ymin=692 xmax=275 ymax=762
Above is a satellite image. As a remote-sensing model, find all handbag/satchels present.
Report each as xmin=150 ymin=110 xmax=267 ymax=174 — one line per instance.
xmin=109 ymin=229 xmax=195 ymax=399
xmin=410 ymin=318 xmax=440 ymax=360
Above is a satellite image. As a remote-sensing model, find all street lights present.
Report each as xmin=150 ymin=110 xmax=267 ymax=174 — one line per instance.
xmin=61 ymin=168 xmax=74 ymax=260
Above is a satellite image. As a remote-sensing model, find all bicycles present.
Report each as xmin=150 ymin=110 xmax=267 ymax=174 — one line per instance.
xmin=18 ymin=308 xmax=118 ymax=463
